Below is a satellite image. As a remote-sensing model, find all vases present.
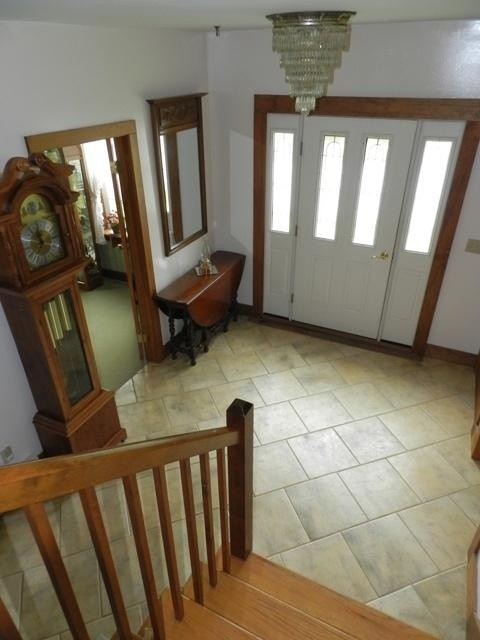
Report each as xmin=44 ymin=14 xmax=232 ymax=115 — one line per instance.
xmin=111 ymin=224 xmax=120 ymax=234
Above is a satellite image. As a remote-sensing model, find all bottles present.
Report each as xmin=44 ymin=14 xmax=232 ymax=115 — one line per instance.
xmin=200 ymin=258 xmax=212 ymax=274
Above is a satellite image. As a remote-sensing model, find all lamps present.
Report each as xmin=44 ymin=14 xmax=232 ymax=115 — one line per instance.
xmin=264 ymin=10 xmax=356 ymax=123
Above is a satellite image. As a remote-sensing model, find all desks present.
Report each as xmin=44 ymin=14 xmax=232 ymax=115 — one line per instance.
xmin=153 ymin=249 xmax=246 ymax=365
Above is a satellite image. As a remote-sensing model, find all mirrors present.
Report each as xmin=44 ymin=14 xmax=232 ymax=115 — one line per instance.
xmin=146 ymin=91 xmax=210 ymax=259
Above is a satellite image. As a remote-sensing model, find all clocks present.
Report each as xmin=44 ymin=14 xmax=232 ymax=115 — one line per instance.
xmin=13 ymin=215 xmax=70 ymax=273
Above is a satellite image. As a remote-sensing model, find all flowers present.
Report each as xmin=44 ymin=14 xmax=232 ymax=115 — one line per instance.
xmin=103 ymin=210 xmax=120 ymax=224
